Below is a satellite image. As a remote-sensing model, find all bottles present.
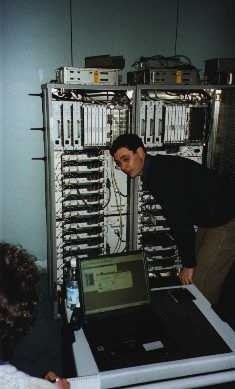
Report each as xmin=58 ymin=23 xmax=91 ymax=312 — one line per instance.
xmin=66 ymin=259 xmax=80 ymax=308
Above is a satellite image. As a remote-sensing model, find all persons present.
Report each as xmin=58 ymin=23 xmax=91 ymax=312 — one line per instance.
xmin=0 ymin=241 xmax=70 ymax=389
xmin=110 ymin=133 xmax=234 ymax=305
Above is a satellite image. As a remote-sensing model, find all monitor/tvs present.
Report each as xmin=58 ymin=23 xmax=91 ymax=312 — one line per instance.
xmin=78 ymin=250 xmax=153 ymax=321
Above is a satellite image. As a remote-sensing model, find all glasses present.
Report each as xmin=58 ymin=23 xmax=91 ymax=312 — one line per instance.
xmin=115 ymin=152 xmax=135 ymax=170
